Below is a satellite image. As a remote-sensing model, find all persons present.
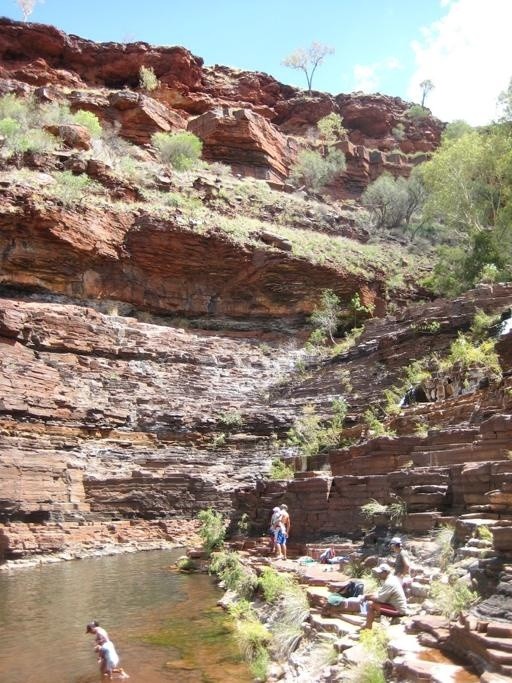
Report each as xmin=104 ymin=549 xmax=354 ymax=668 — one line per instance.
xmin=83 ymin=618 xmax=112 ymax=678
xmin=355 ymin=561 xmax=410 ymax=632
xmin=267 ymin=510 xmax=282 ymax=554
xmin=95 ymin=634 xmax=125 ymax=679
xmin=320 ymin=542 xmax=335 ymax=563
xmin=271 ymin=505 xmax=283 ymax=531
xmin=379 ymin=535 xmax=411 ymax=590
xmin=271 ymin=502 xmax=292 ymax=560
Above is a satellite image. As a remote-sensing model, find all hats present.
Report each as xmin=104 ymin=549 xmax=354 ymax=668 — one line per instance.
xmin=86 ymin=624 xmax=96 ymax=633
xmin=372 ymin=564 xmax=390 ymax=572
xmin=391 ymin=537 xmax=401 ymax=544
